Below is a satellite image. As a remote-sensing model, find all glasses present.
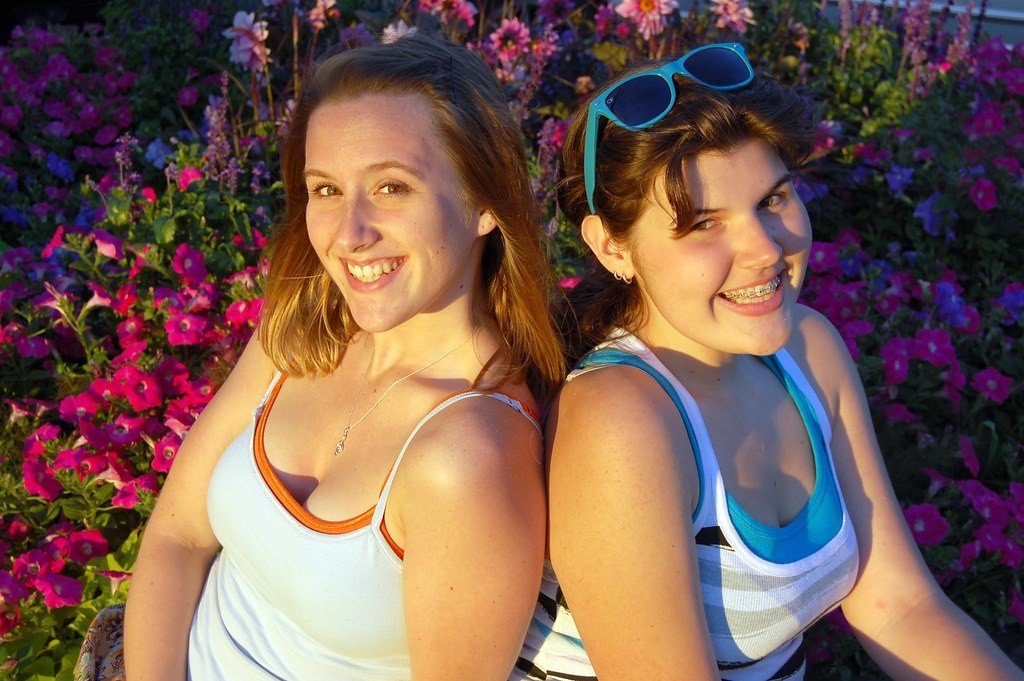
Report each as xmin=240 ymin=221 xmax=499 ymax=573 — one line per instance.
xmin=584 ymin=42 xmax=754 ymax=215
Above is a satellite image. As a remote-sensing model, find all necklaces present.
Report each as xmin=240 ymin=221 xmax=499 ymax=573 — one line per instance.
xmin=331 ymin=323 xmax=479 ymax=455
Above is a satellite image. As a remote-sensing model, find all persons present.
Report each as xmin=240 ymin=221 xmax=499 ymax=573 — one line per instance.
xmin=505 ymin=40 xmax=1024 ymax=681
xmin=71 ymin=34 xmax=575 ymax=681
